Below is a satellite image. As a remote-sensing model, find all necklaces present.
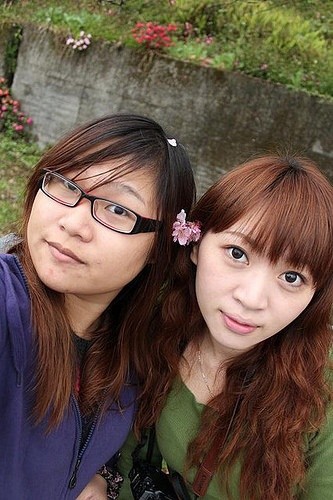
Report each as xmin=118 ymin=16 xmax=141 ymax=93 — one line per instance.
xmin=195 ymin=340 xmax=216 ymax=400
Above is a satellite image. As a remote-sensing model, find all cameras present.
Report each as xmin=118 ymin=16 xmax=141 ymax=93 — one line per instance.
xmin=129 ymin=462 xmax=191 ymax=500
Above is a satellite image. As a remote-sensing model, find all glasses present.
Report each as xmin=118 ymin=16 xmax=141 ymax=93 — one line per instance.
xmin=38 ymin=166 xmax=163 ymax=236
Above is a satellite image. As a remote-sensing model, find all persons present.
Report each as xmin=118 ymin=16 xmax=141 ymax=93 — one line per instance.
xmin=1 ymin=105 xmax=199 ymax=500
xmin=118 ymin=150 xmax=332 ymax=500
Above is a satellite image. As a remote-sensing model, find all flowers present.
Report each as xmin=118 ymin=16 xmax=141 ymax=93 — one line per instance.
xmin=172 ymin=208 xmax=202 ymax=246
